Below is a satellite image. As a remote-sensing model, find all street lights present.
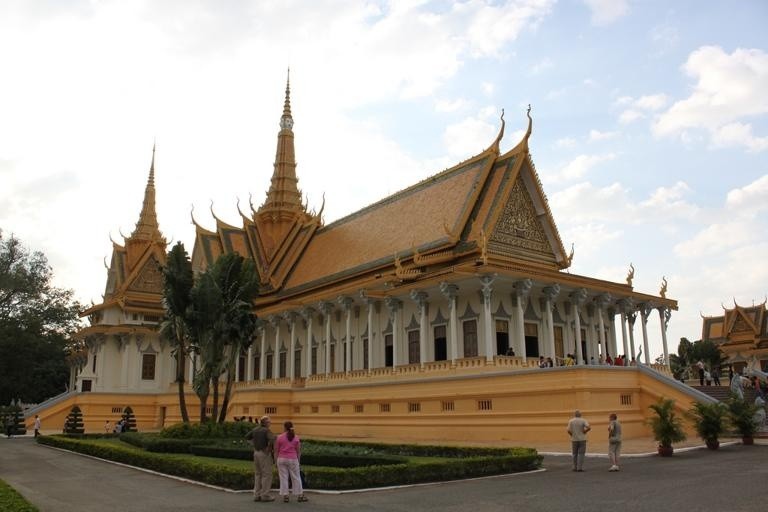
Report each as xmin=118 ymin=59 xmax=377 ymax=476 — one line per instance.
xmin=609 ymin=430 xmax=615 ymax=436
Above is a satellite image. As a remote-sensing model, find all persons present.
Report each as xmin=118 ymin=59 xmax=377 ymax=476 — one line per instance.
xmin=673 ymin=357 xmax=768 ymax=404
xmin=104 ymin=420 xmax=110 ymax=432
xmin=537 ymin=353 xmax=638 ymax=368
xmin=33 ymin=414 xmax=40 ymax=438
xmin=113 ymin=420 xmax=125 ymax=435
xmin=607 ymin=413 xmax=623 ymax=472
xmin=244 ymin=416 xmax=277 ymax=501
xmin=507 ymin=348 xmax=515 ymax=355
xmin=276 ymin=421 xmax=308 ymax=502
xmin=566 ymin=410 xmax=590 ymax=472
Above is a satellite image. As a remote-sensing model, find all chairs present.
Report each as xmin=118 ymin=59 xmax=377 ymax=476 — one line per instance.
xmin=283 ymin=496 xmax=290 ymax=503
xmin=254 ymin=495 xmax=274 ymax=502
xmin=608 ymin=463 xmax=619 ymax=470
xmin=298 ymin=495 xmax=309 ymax=500
xmin=573 ymin=468 xmax=582 ymax=471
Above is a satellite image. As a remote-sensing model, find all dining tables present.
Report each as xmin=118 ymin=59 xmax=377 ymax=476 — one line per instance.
xmin=645 ymin=397 xmax=761 ymax=457
